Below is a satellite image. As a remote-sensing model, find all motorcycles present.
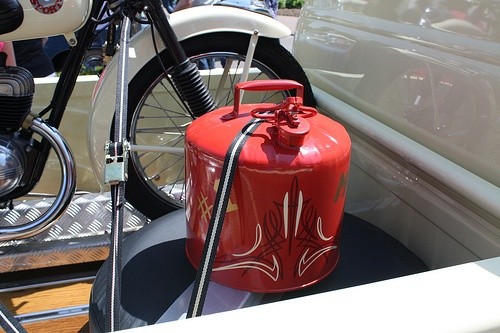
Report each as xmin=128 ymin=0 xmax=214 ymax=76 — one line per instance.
xmin=0 ymin=1 xmax=316 ymax=256
